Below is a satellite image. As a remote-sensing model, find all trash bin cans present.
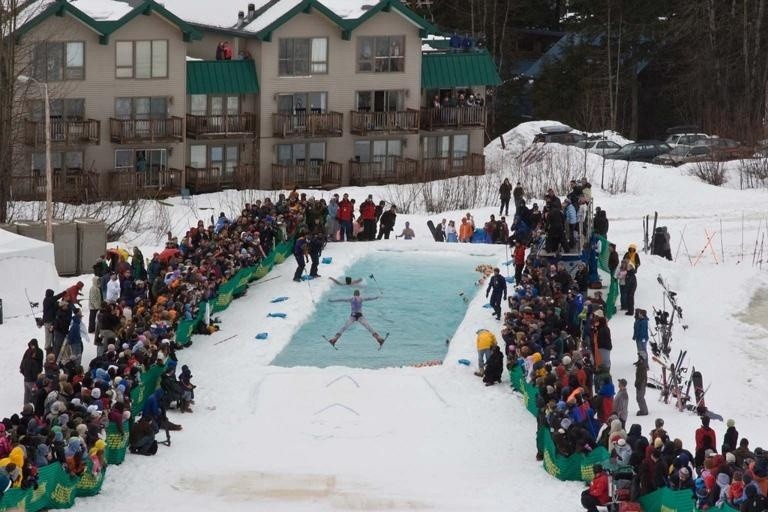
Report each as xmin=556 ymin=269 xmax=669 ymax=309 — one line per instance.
xmin=0 ymin=217 xmax=106 ymax=276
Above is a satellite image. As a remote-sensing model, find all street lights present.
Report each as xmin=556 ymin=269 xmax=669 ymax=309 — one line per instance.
xmin=15 ymin=73 xmax=53 ymax=242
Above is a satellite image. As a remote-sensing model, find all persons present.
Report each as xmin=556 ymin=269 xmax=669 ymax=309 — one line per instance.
xmin=474 ymin=329 xmax=498 ymax=376
xmin=215 ymin=40 xmax=250 ymax=59
xmin=499 ymin=178 xmax=513 ymax=215
xmin=376 ymin=38 xmax=400 ymax=69
xmin=504 ymin=178 xmax=608 ymax=343
xmin=662 ymin=226 xmax=673 ymax=261
xmin=633 ymin=352 xmax=647 ymax=416
xmin=293 ymin=234 xmax=307 ymax=282
xmin=136 ymin=152 xmax=145 ymax=170
xmin=501 ymin=326 xmax=627 ymax=461
xmin=220 ymin=191 xmax=330 ymax=271
xmin=632 ymin=309 xmax=649 ymax=364
xmin=445 ymin=29 xmax=488 ymax=52
xmin=1 ymin=339 xmax=196 ymax=511
xmin=396 ymin=222 xmax=415 ymax=240
xmin=650 ymin=227 xmax=667 ymax=258
xmin=608 ymin=243 xmax=641 ymax=316
xmin=41 ymin=280 xmax=84 ymax=354
xmin=431 ymin=90 xmax=483 ymax=107
xmin=482 ymin=344 xmax=504 ymax=387
xmin=308 ymin=231 xmax=325 ymax=278
xmin=435 ymin=213 xmax=509 ymax=244
xmin=88 ymin=221 xmax=267 ymax=355
xmin=486 ymin=268 xmax=508 ymax=320
xmin=580 ymin=414 xmax=768 ymax=512
xmin=329 ymin=277 xmax=362 ymax=285
xmin=328 ymin=290 xmax=384 ymax=346
xmin=328 ymin=192 xmax=398 ymax=241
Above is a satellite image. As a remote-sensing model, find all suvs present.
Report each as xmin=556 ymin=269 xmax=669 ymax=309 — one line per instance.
xmin=531 ymin=123 xmax=583 ymax=147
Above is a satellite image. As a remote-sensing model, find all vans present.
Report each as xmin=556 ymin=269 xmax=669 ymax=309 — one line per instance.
xmin=667 ymin=133 xmax=708 ymax=145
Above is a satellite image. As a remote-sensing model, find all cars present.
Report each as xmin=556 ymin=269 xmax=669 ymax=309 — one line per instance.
xmin=579 ymin=138 xmax=754 ymax=166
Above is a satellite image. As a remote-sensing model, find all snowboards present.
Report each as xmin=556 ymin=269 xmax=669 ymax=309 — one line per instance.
xmin=693 ymin=371 xmax=707 ymax=417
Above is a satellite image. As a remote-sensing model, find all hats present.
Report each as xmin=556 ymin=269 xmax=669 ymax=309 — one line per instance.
xmin=561 ymin=356 xmax=765 ymax=496
xmin=36 ymin=339 xmax=170 ymax=455
